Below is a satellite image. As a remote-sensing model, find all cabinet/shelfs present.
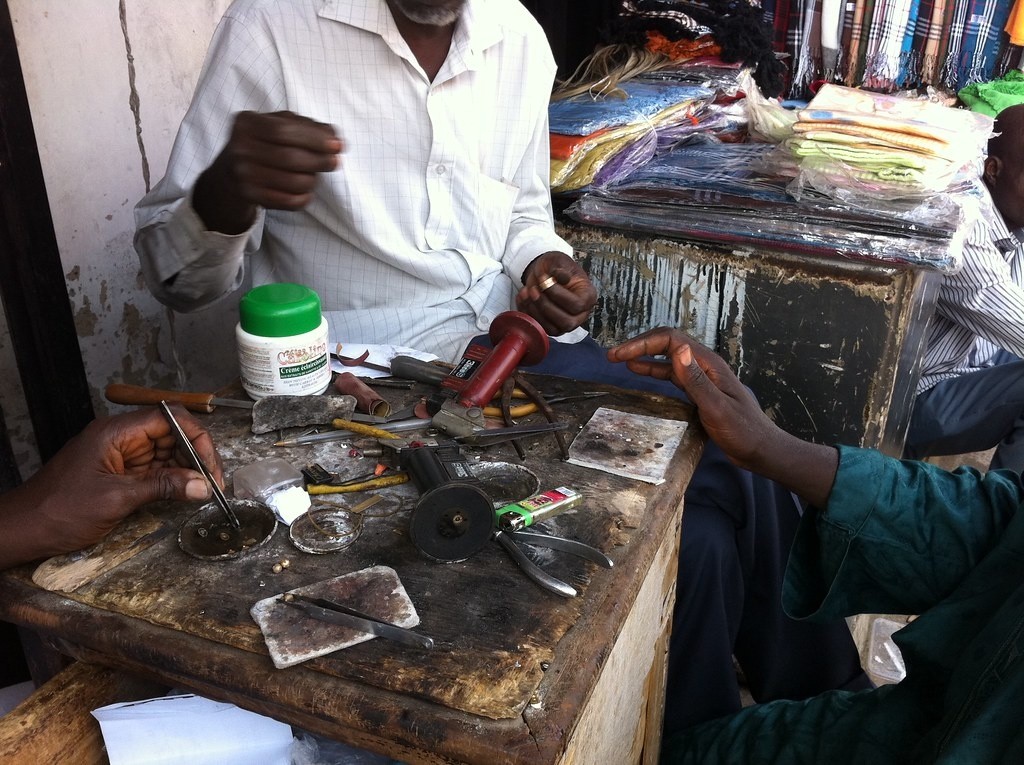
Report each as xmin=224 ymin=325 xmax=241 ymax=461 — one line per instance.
xmin=552 ymin=193 xmax=963 ymax=462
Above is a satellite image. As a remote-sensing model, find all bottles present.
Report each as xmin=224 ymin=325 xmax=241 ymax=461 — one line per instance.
xmin=234 ymin=283 xmax=332 ymax=402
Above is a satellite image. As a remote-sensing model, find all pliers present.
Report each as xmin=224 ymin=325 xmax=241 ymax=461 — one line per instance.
xmin=482 ymin=388 xmax=610 ymax=418
xmin=503 ymin=368 xmax=571 ymax=461
xmin=494 ymin=528 xmax=614 ymax=599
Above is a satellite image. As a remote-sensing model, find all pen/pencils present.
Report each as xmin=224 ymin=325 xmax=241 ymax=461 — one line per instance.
xmin=273 ymin=418 xmax=433 ymax=446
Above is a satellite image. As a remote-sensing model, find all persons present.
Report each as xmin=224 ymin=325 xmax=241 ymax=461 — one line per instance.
xmin=0 ymin=401 xmax=229 ymax=566
xmin=606 ymin=322 xmax=1024 ymax=765
xmin=133 ymin=0 xmax=876 ymax=717
xmin=905 ymin=101 xmax=1024 ymax=475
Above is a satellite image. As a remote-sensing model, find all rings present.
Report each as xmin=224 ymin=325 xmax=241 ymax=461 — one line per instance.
xmin=538 ymin=276 xmax=558 ymax=293
